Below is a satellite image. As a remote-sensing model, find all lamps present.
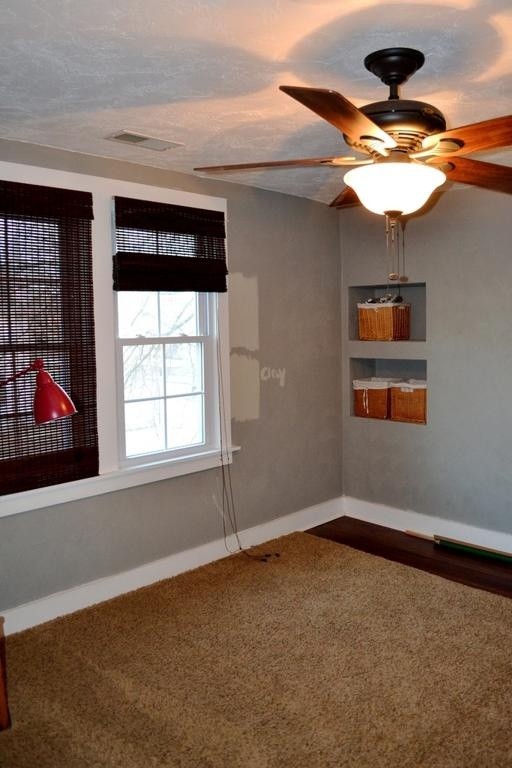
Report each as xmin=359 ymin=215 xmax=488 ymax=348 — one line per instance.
xmin=1 ymin=360 xmax=80 ymax=427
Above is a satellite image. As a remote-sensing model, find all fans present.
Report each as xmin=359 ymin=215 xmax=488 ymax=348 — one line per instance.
xmin=191 ymin=46 xmax=512 ymax=210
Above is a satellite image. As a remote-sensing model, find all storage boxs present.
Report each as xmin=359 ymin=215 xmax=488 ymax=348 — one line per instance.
xmin=352 ymin=376 xmax=426 ymax=424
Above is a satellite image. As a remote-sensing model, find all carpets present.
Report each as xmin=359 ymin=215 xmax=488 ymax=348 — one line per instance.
xmin=1 ymin=531 xmax=512 ymax=768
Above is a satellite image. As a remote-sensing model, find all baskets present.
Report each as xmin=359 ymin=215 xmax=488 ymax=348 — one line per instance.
xmin=352 ymin=376 xmax=426 ymax=424
